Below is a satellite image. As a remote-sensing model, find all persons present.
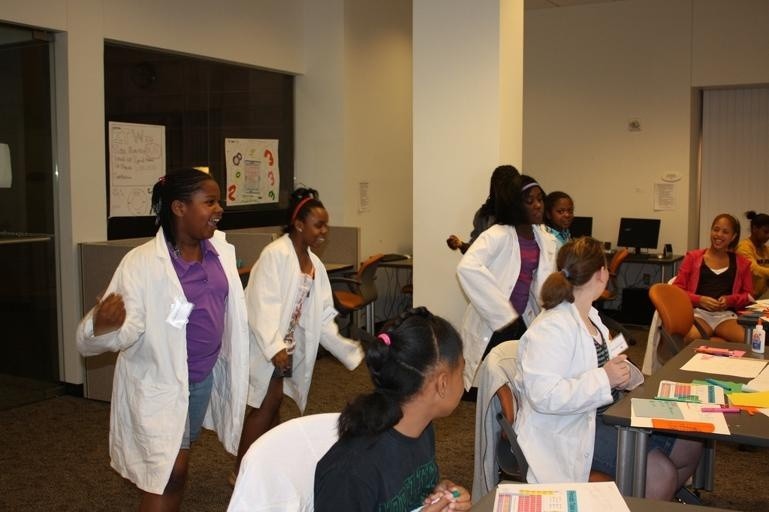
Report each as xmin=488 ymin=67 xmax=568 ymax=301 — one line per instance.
xmin=447 ymin=165 xmax=520 ymax=256
xmin=226 ymin=185 xmax=367 ymax=490
xmin=734 ymin=210 xmax=769 ymax=300
xmin=71 ymin=165 xmax=251 ymax=512
xmin=543 ymin=190 xmax=577 ymax=248
xmin=511 ymin=235 xmax=705 ymax=502
xmin=456 ymin=173 xmax=567 ymax=393
xmin=672 ymin=212 xmax=753 ymax=351
xmin=313 ymin=305 xmax=472 ymax=512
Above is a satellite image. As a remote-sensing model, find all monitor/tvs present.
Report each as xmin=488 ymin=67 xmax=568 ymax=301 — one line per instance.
xmin=570 ymin=216 xmax=593 ymax=238
xmin=617 ymin=217 xmax=660 ymax=259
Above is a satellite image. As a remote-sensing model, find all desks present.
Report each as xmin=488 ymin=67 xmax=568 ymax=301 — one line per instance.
xmin=236 ymin=261 xmax=259 ymax=275
xmin=608 ymin=252 xmax=685 ymax=285
xmin=735 ymin=288 xmax=768 ymax=347
xmin=601 ymin=335 xmax=769 ymax=504
xmin=378 ymin=258 xmax=412 ymax=322
xmin=464 ymin=478 xmax=726 ymax=511
xmin=320 ymin=262 xmax=351 ymax=273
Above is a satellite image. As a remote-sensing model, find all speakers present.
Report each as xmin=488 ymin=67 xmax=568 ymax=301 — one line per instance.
xmin=663 ymin=243 xmax=673 ymax=258
xmin=604 ymin=242 xmax=611 ymax=254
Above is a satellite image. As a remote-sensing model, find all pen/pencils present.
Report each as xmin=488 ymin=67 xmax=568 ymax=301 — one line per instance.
xmin=706 ymin=378 xmax=731 ymax=391
xmin=702 ymin=408 xmax=740 ymax=412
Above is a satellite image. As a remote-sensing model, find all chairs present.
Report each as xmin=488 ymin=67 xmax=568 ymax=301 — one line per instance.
xmin=473 ymin=339 xmax=611 ymax=483
xmin=646 ymin=282 xmax=701 ymax=367
xmin=224 ymin=410 xmax=346 ymax=512
xmin=331 ymin=251 xmax=386 ymax=335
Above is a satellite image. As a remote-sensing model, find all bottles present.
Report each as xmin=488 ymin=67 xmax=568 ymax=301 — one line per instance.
xmin=751 ymin=320 xmax=766 ymax=353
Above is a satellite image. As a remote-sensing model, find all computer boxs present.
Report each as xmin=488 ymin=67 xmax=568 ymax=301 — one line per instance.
xmin=621 ymin=287 xmax=656 ymax=325
